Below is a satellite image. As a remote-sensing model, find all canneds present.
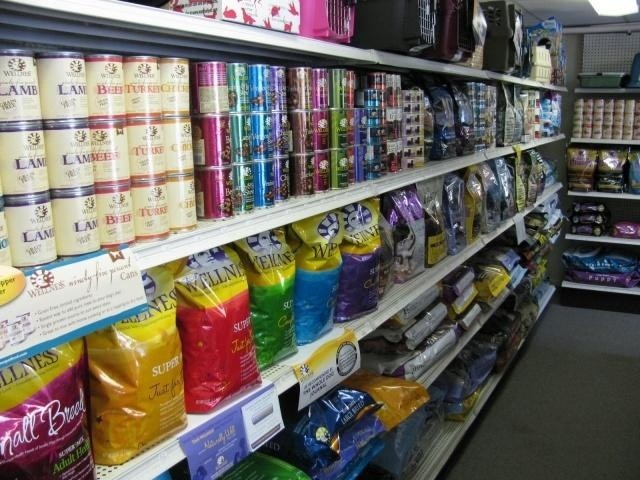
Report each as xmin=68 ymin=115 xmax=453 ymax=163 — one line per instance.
xmin=0 ymin=46 xmax=497 ymax=269
xmin=572 ymin=98 xmax=640 ymax=140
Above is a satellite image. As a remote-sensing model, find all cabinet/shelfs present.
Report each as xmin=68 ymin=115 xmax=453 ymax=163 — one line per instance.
xmin=558 ymin=21 xmax=640 ymax=298
xmin=0 ymin=2 xmax=572 ymax=480
